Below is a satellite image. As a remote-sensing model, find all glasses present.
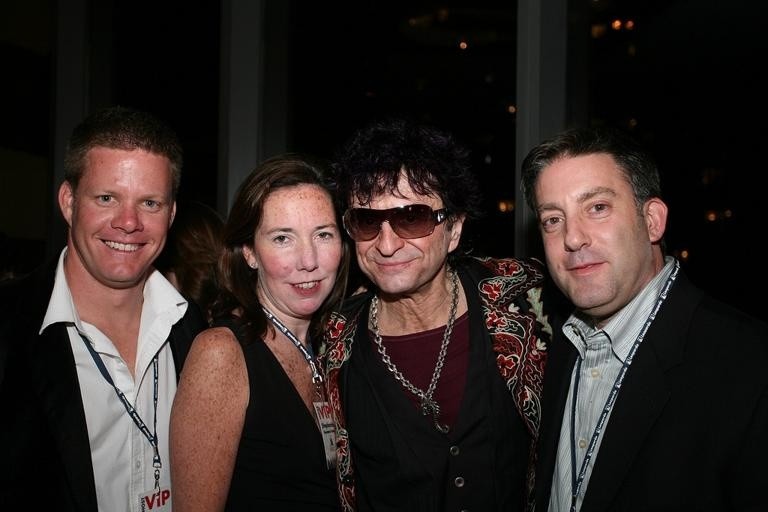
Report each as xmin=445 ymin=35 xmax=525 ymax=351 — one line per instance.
xmin=342 ymin=205 xmax=447 ymax=241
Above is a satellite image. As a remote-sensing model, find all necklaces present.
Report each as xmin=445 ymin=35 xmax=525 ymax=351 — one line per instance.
xmin=370 ymin=268 xmax=459 ymax=434
xmin=261 ymin=303 xmax=341 ymax=470
xmin=72 ymin=326 xmax=162 ymax=497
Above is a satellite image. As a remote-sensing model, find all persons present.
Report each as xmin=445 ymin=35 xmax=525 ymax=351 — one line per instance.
xmin=153 ymin=200 xmax=228 ymax=332
xmin=519 ymin=118 xmax=767 ymax=511
xmin=153 ymin=156 xmax=352 ymax=510
xmin=2 ymin=107 xmax=206 ymax=510
xmin=312 ymin=113 xmax=555 ymax=509
